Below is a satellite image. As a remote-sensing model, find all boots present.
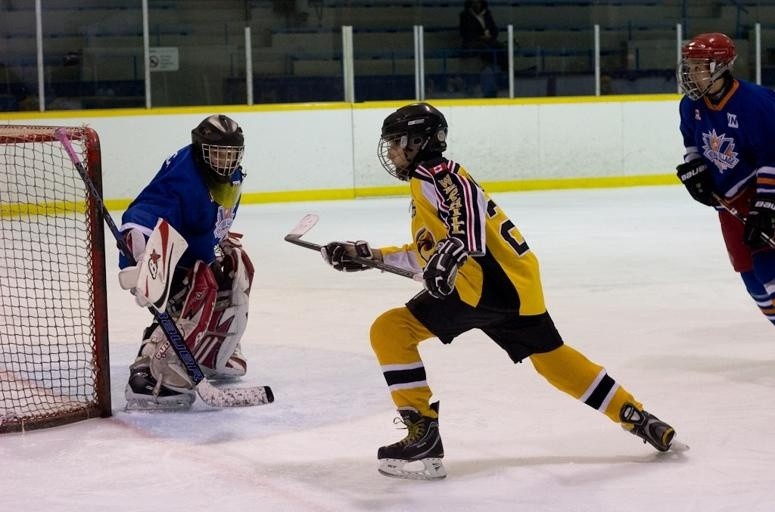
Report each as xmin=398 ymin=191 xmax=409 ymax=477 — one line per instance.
xmin=619 ymin=402 xmax=675 ymax=452
xmin=377 ymin=400 xmax=445 ymax=462
xmin=125 ymin=324 xmax=247 ymax=404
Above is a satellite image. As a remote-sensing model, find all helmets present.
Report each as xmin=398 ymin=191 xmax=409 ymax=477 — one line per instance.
xmin=681 ymin=32 xmax=736 ymax=64
xmin=191 ymin=114 xmax=244 ymax=183
xmin=381 ymin=102 xmax=448 ymax=152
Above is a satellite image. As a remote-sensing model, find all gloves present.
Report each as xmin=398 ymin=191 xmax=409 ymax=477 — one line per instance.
xmin=118 ymin=217 xmax=189 ymax=314
xmin=676 ymin=157 xmax=721 ymax=206
xmin=321 ymin=239 xmax=384 ymax=273
xmin=422 ymin=237 xmax=468 ymax=299
xmin=743 ymin=197 xmax=775 ymax=248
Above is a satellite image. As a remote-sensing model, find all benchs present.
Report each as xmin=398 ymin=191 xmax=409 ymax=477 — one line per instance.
xmin=1 ymin=0 xmax=775 ymax=101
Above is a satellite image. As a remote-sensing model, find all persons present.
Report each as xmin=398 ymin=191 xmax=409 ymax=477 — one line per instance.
xmin=458 ymin=0 xmax=510 ymax=91
xmin=117 ymin=114 xmax=253 ymax=404
xmin=320 ymin=101 xmax=689 ymax=462
xmin=675 ymin=33 xmax=775 ymax=329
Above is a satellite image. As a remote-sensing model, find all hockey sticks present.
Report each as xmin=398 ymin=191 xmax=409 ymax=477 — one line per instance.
xmin=54 ymin=127 xmax=275 ymax=407
xmin=284 ymin=213 xmax=425 ymax=285
